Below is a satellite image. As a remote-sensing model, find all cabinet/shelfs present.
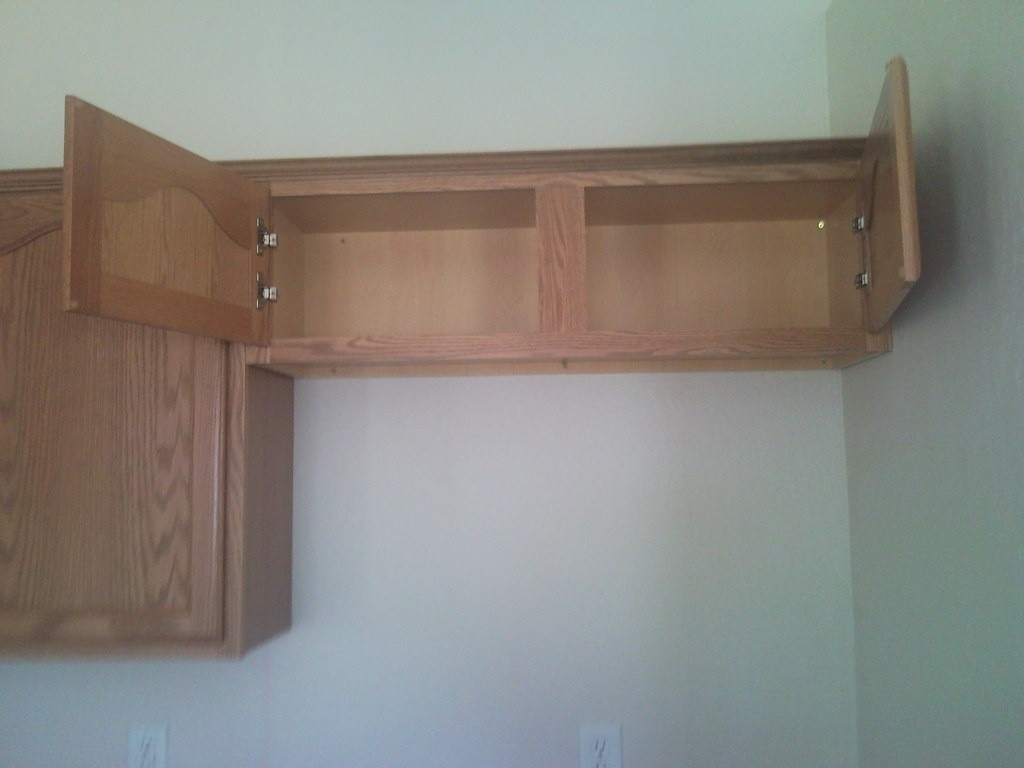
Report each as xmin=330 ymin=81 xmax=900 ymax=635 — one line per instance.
xmin=0 ymin=56 xmax=923 ymax=661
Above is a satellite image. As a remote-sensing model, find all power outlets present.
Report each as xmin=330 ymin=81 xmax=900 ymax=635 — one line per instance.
xmin=579 ymin=721 xmax=622 ymax=768
xmin=126 ymin=720 xmax=168 ymax=768
